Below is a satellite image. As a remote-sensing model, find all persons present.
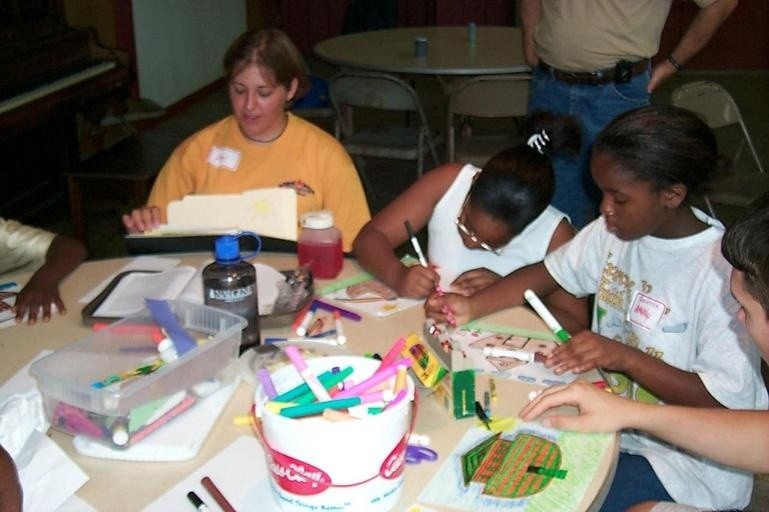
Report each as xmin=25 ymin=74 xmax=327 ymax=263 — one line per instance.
xmin=518 ymin=0 xmax=739 ymax=232
xmin=424 ymin=101 xmax=769 ymax=512
xmin=121 ymin=26 xmax=373 ymax=256
xmin=0 ymin=218 xmax=88 ymax=326
xmin=518 ymin=202 xmax=769 ymax=512
xmin=348 ymin=112 xmax=591 ymax=337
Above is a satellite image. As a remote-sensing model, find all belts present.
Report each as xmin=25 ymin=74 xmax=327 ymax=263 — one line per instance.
xmin=535 ymin=55 xmax=668 ymax=89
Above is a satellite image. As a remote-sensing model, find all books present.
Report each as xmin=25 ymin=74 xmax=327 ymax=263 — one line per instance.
xmin=93 ymin=258 xmax=288 ymax=322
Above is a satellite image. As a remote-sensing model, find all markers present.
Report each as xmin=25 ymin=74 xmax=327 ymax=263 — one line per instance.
xmin=314 ymin=299 xmax=361 ymax=322
xmin=292 ymin=297 xmax=314 ymax=330
xmin=476 ymin=401 xmax=492 ymax=431
xmin=484 ymin=345 xmax=547 ymax=365
xmin=489 ymin=379 xmax=497 ymax=401
xmin=187 ymin=492 xmax=212 ymax=512
xmin=306 ymin=318 xmax=323 ymax=336
xmin=405 ymin=220 xmax=428 ymax=268
xmin=524 ymin=290 xmax=570 ymax=343
xmin=435 ymin=285 xmax=456 ymax=328
xmin=233 ymin=338 xmax=412 ymax=427
xmin=313 ymin=330 xmax=335 ymax=338
xmin=297 ymin=303 xmax=318 ymax=336
xmin=202 ymin=477 xmax=234 ymax=512
xmin=484 ymin=393 xmax=490 ymax=417
xmin=334 ymin=311 xmax=346 ymax=344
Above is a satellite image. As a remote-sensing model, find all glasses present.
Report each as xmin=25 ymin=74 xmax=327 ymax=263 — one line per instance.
xmin=452 ymin=193 xmax=514 ymax=257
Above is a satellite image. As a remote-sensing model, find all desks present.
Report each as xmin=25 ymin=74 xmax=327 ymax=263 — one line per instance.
xmin=313 ymin=24 xmax=537 ymax=75
xmin=1 ymin=248 xmax=622 ymax=511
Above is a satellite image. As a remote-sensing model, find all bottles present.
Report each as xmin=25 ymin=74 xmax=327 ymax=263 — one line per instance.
xmin=203 ymin=231 xmax=262 ymax=358
xmin=297 ymin=211 xmax=344 ymax=279
xmin=461 ymin=120 xmax=472 ymax=139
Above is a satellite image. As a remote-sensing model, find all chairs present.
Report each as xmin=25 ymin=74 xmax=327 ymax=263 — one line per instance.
xmin=671 ymin=80 xmax=767 ymax=222
xmin=326 ymin=73 xmax=443 ymax=196
xmin=442 ymin=75 xmax=534 ymax=165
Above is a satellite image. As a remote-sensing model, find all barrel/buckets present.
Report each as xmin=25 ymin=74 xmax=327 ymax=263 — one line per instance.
xmin=247 ymin=354 xmax=418 ymax=512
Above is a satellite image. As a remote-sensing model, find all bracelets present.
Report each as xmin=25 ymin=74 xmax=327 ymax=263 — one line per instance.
xmin=667 ymin=53 xmax=682 ymax=73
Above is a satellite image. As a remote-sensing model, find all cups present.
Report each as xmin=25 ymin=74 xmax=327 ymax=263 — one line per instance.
xmin=415 ymin=37 xmax=428 ymax=57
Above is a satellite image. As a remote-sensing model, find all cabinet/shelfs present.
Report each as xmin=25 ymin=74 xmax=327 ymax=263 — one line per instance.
xmin=0 ymin=57 xmax=166 ymax=240
